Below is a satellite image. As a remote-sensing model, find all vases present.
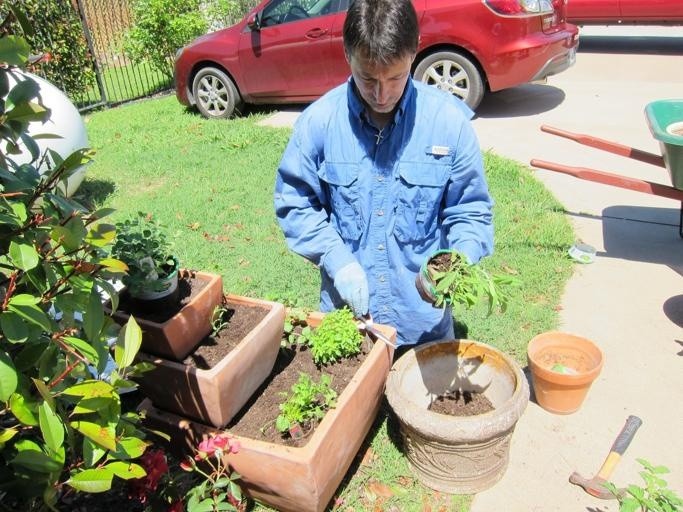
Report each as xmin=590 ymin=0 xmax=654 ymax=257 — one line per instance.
xmin=383 ymin=339 xmax=526 ymax=493
xmin=525 ymin=332 xmax=606 ymax=416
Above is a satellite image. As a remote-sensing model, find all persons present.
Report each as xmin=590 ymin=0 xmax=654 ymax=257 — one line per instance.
xmin=273 ymin=0 xmax=496 ymax=355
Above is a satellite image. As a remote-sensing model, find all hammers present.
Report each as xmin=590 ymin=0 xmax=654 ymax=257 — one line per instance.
xmin=570 ymin=415 xmax=641 ymax=499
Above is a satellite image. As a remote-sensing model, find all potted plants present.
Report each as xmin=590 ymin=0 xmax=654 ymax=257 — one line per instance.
xmin=418 ymin=250 xmax=521 ymax=315
xmin=111 ymin=288 xmax=285 ymax=425
xmin=98 ymin=266 xmax=224 ymax=359
xmin=113 ymin=213 xmax=181 ymax=296
xmin=139 ymin=310 xmax=397 ymax=511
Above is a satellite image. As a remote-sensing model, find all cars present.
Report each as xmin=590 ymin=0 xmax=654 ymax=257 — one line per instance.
xmin=172 ymin=1 xmax=580 ymax=113
xmin=566 ymin=1 xmax=681 ymax=27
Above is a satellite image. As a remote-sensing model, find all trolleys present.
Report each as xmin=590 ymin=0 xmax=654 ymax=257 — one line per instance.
xmin=533 ymin=98 xmax=683 ymax=235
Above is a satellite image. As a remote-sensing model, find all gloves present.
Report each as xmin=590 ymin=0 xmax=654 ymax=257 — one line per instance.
xmin=323 ymin=244 xmax=369 ymax=320
